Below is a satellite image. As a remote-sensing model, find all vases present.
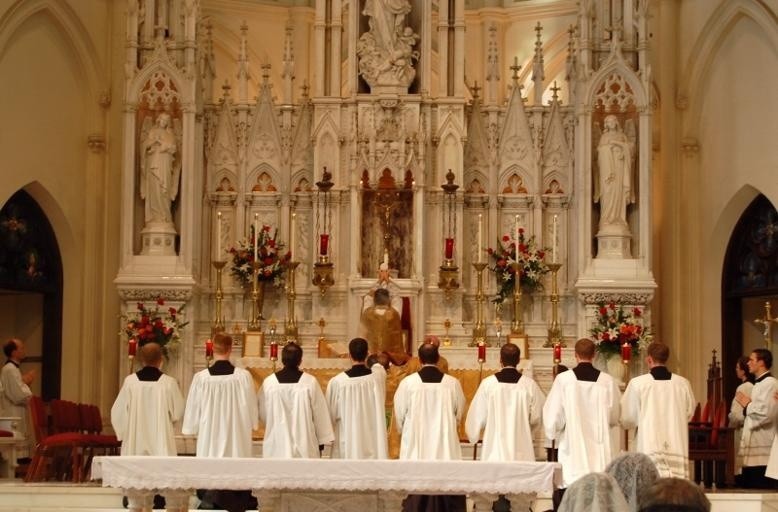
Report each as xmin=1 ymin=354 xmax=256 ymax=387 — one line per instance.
xmin=606 ymin=351 xmax=626 ymax=383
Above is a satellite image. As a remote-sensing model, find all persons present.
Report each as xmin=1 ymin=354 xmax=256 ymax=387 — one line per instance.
xmin=728 ymin=349 xmax=777 ymax=491
xmin=597 ymin=115 xmax=632 ymax=226
xmin=362 ymin=269 xmax=403 ymax=367
xmin=393 ymin=337 xmax=712 ymax=511
xmin=0 ymin=338 xmax=36 ymax=478
xmin=111 ymin=332 xmax=387 ymax=509
xmin=362 ymin=0 xmax=416 ymax=71
xmin=143 ymin=113 xmax=177 ymax=222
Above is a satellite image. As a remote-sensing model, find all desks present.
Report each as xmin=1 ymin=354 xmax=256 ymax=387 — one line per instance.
xmin=90 ymin=452 xmax=565 ymax=512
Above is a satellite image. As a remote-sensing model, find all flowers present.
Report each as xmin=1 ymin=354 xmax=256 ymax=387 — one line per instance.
xmin=481 ymin=227 xmax=553 ymax=305
xmin=589 ymin=297 xmax=656 ymax=350
xmin=116 ymin=295 xmax=189 ymax=362
xmin=223 ymin=223 xmax=291 ymax=287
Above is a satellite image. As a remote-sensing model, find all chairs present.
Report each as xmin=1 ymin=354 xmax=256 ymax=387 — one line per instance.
xmin=23 ymin=396 xmax=122 ymax=484
xmin=687 ymin=401 xmax=735 ymax=489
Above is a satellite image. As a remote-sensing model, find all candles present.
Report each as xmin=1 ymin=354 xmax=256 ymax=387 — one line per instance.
xmin=553 ymin=342 xmax=561 ymax=360
xmin=446 ymin=236 xmax=454 ymax=257
xmin=254 ymin=213 xmax=259 ymax=262
xmin=478 ymin=341 xmax=485 ymax=359
xmin=206 ymin=339 xmax=214 ymax=355
xmin=478 ymin=213 xmax=482 ymax=261
xmin=412 ymin=181 xmax=416 ymax=188
xmin=291 ymin=214 xmax=296 ymax=261
xmin=217 ymin=212 xmax=222 ymax=261
xmin=320 ymin=233 xmax=330 ymax=255
xmin=270 ymin=341 xmax=278 ymax=357
xmin=359 ymin=180 xmax=364 ymax=188
xmin=622 ymin=343 xmax=632 ymax=359
xmin=383 ymin=251 xmax=389 ymax=262
xmin=515 ymin=215 xmax=519 ymax=262
xmin=128 ymin=340 xmax=135 ymax=356
xmin=552 ymin=215 xmax=557 ymax=263
